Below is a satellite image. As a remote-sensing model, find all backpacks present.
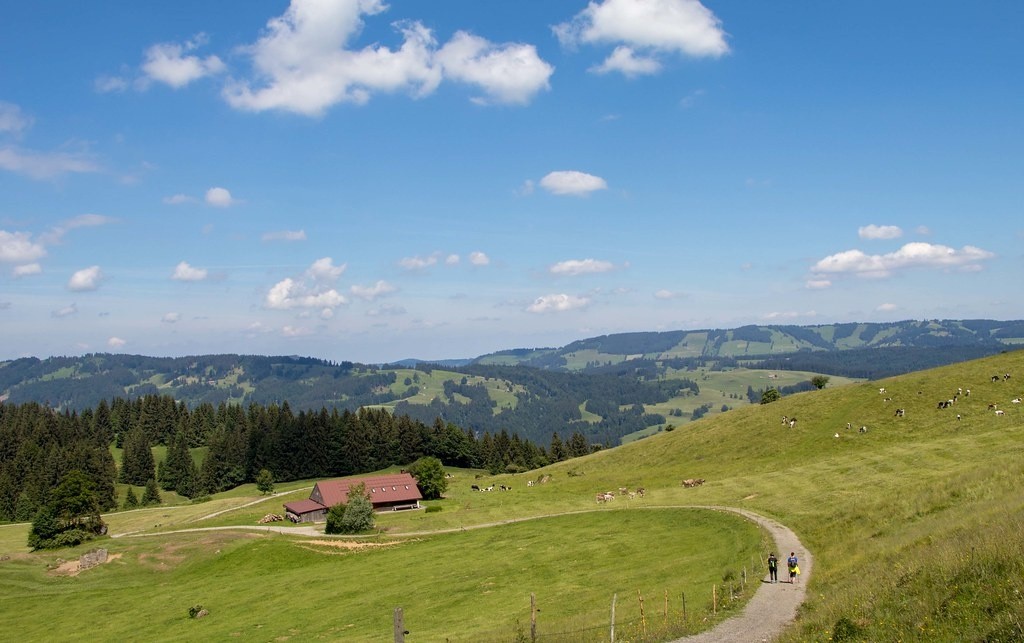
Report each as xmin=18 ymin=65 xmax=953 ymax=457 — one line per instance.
xmin=769 ymin=559 xmax=775 ymax=568
xmin=790 ymin=558 xmax=795 ymax=568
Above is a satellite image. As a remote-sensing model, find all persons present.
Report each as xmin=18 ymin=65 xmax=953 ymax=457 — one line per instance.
xmin=768 ymin=553 xmax=778 ymax=584
xmin=787 ymin=552 xmax=798 ymax=583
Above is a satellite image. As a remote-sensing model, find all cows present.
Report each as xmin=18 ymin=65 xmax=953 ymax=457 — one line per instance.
xmin=472 ymin=479 xmax=536 ymax=492
xmin=936 ymin=388 xmax=971 ymax=409
xmin=879 ymin=387 xmax=904 ymax=418
xmin=917 ymin=391 xmax=923 ymax=395
xmin=956 ymin=397 xmax=1023 ymax=421
xmin=846 ymin=422 xmax=867 ymax=433
xmin=596 ymin=486 xmax=645 ymax=502
xmin=781 ymin=415 xmax=799 ymax=428
xmin=991 ymin=373 xmax=1010 ymax=382
xmin=682 ymin=478 xmax=706 ymax=487
